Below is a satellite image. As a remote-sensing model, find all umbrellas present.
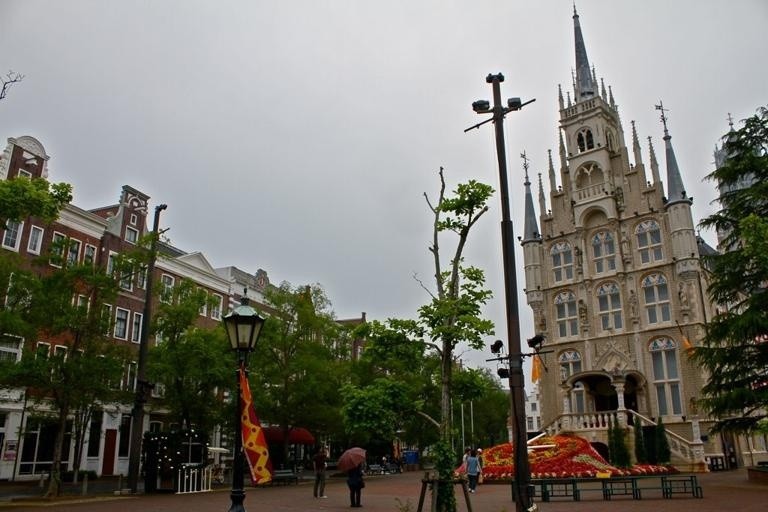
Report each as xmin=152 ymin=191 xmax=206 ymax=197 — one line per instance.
xmin=336 ymin=447 xmax=366 ymax=472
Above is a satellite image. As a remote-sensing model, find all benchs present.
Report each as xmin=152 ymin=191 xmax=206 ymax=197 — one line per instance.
xmin=661 ymin=476 xmax=703 ymax=499
xmin=263 ymin=469 xmax=297 ymax=488
xmin=602 ymin=479 xmax=642 ymax=501
xmin=530 ymin=477 xmax=580 ymax=501
xmin=369 ymin=465 xmax=386 ymax=476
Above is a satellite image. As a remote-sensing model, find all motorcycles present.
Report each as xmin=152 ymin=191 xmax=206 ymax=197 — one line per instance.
xmin=380 ymin=455 xmax=405 ymax=475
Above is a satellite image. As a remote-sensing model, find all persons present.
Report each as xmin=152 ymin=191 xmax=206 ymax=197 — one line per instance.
xmin=311 ymin=445 xmax=327 ymax=498
xmin=476 ymin=448 xmax=483 ymax=469
xmin=464 ymin=450 xmax=482 ymax=494
xmin=347 ymin=463 xmax=365 ymax=508
xmin=463 ymin=448 xmax=470 ymax=465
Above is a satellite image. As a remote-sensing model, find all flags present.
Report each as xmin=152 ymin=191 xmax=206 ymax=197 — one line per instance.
xmin=532 ymin=355 xmax=542 ymax=383
xmin=682 ymin=335 xmax=694 ymax=355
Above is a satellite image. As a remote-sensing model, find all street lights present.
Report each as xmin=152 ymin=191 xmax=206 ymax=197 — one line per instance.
xmin=462 ymin=73 xmax=553 ymax=511
xmin=222 ymin=285 xmax=265 ymax=512
